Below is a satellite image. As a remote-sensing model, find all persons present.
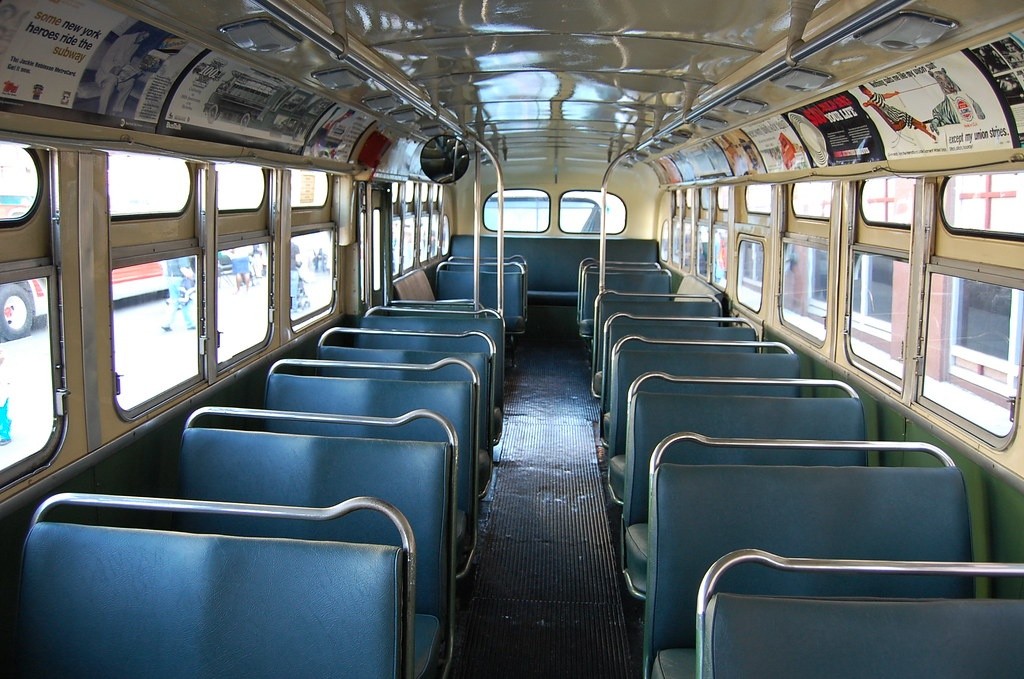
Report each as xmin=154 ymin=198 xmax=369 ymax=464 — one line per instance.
xmin=160 ymin=258 xmax=197 ymax=332
xmin=95 ymin=31 xmax=151 ymax=116
xmin=0 ymin=349 xmax=16 ymax=445
xmin=229 ymin=243 xmax=330 ymax=313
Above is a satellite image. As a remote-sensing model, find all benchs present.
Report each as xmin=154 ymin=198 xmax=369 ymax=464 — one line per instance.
xmin=392 ymin=268 xmax=488 ymax=318
xmin=695 ymin=548 xmax=1024 ymax=679
xmin=314 ymin=327 xmax=496 ymax=498
xmin=644 ymin=432 xmax=975 ymax=679
xmin=353 ymin=306 xmax=504 ymax=447
xmin=599 ymin=312 xmax=762 ymax=449
xmin=673 ymin=273 xmax=723 ymax=302
xmin=576 ymin=257 xmax=662 ymax=362
xmin=608 ymin=333 xmax=799 ymax=504
xmin=448 ymin=234 xmax=658 ymax=304
xmin=10 ymin=495 xmax=417 ymax=679
xmin=447 ymin=255 xmax=528 ymax=352
xmin=261 ymin=356 xmax=481 ymax=580
xmin=620 ymin=370 xmax=868 ymax=601
xmin=171 ymin=406 xmax=460 ymax=679
xmin=579 ymin=263 xmax=673 ymax=371
xmin=435 ymin=262 xmax=525 ymax=367
